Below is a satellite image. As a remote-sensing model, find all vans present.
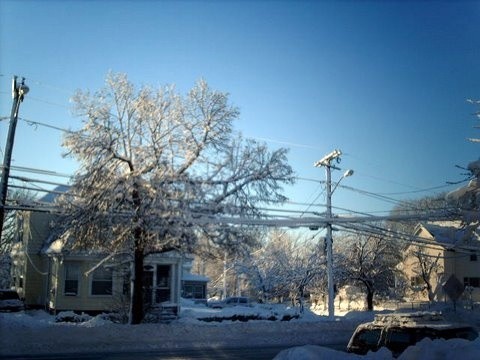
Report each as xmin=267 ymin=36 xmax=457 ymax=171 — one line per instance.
xmin=0 ymin=289 xmax=25 ymax=313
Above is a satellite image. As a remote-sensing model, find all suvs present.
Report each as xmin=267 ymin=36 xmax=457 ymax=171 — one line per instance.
xmin=346 ymin=311 xmax=480 ymax=359
xmin=209 ymin=296 xmax=253 ymax=309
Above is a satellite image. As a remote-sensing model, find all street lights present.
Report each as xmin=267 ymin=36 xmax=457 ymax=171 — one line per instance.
xmin=0 ymin=85 xmax=30 ymax=238
xmin=326 ymin=169 xmax=354 ymax=318
